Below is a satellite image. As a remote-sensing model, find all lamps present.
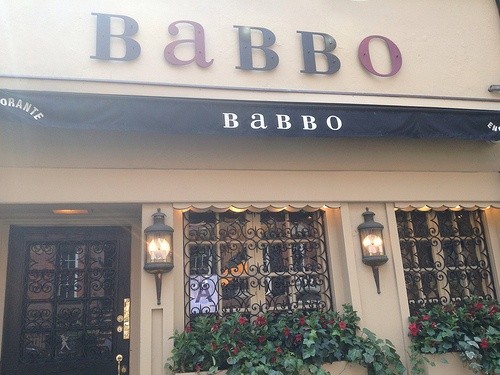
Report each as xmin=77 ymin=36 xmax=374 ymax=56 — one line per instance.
xmin=357 ymin=206 xmax=391 ymax=296
xmin=143 ymin=208 xmax=174 ymax=305
